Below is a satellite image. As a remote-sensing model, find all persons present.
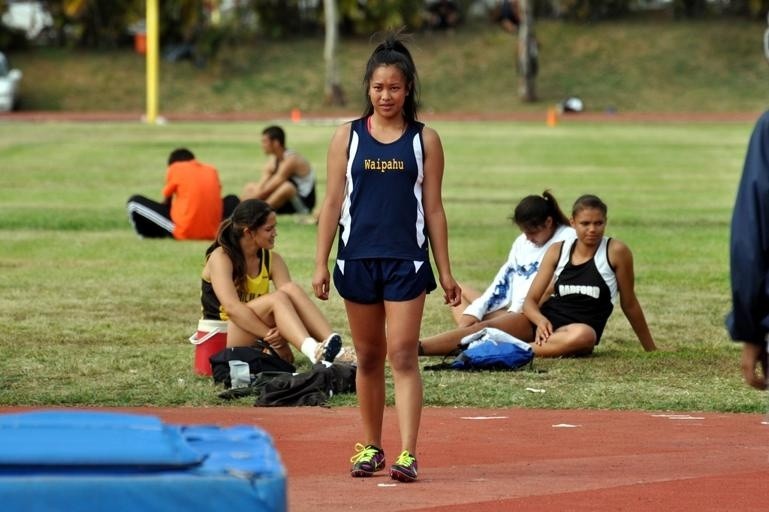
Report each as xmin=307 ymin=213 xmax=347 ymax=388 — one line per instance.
xmin=241 ymin=126 xmax=315 ymax=213
xmin=311 ymin=23 xmax=462 ymax=483
xmin=199 ymin=199 xmax=342 ymax=364
xmin=724 ymin=28 xmax=769 ymax=391
xmin=418 ymin=194 xmax=657 ymax=356
xmin=126 ymin=148 xmax=240 ymax=238
xmin=450 ymin=189 xmax=577 ymax=336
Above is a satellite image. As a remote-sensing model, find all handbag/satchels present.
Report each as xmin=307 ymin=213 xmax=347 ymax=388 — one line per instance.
xmin=427 ymin=343 xmax=538 ymax=376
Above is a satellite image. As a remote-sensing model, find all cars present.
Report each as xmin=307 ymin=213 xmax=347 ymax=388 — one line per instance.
xmin=0 ymin=52 xmax=23 ymax=114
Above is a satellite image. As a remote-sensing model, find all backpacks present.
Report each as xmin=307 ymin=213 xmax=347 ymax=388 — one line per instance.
xmin=209 ymin=341 xmax=297 ymax=385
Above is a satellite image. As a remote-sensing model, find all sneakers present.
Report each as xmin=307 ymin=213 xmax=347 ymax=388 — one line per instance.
xmin=314 ymin=333 xmax=343 ymax=365
xmin=348 ymin=444 xmax=387 ymax=478
xmin=390 ymin=452 xmax=420 ymax=483
